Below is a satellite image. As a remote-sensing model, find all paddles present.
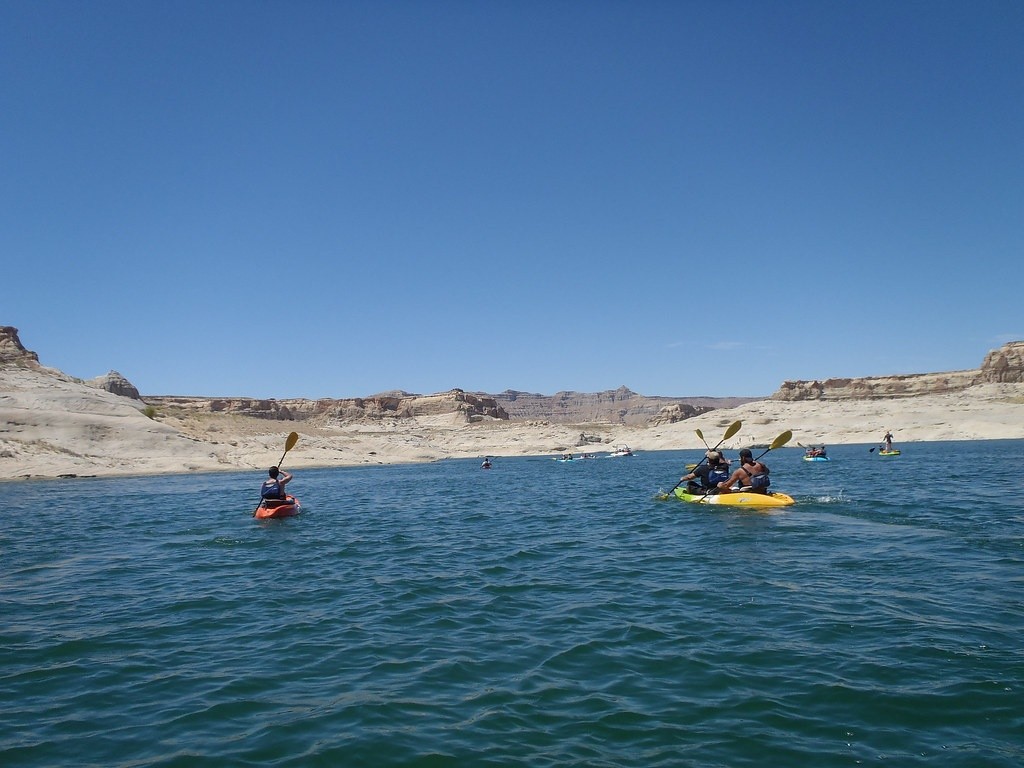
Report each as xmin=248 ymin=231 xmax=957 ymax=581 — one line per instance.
xmin=659 ymin=420 xmax=742 ymax=499
xmin=697 ymin=431 xmax=793 ymax=502
xmin=252 ymin=431 xmax=299 ymax=516
xmin=797 ymin=441 xmax=807 ymax=449
xmin=695 ymin=428 xmax=710 ymax=452
xmin=686 ymin=458 xmax=741 ymax=471
xmin=870 ymin=440 xmax=883 ymax=453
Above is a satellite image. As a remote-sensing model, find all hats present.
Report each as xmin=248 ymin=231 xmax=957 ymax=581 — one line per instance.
xmin=740 ymin=449 xmax=753 ymax=462
xmin=887 ymin=431 xmax=889 ymax=433
xmin=707 ymin=451 xmax=719 ymax=460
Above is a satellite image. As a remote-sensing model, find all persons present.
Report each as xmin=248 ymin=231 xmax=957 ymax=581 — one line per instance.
xmin=481 ymin=458 xmax=492 ymax=468
xmin=884 ymin=431 xmax=894 ymax=452
xmin=560 ymin=453 xmax=590 ymax=462
xmin=681 ymin=450 xmax=732 ymax=497
xmin=260 ymin=466 xmax=293 ymax=509
xmin=805 ymin=445 xmax=827 ymax=458
xmin=718 ymin=449 xmax=770 ymax=494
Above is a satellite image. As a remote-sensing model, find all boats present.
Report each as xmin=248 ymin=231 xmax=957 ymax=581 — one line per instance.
xmin=685 ymin=464 xmax=699 ymax=471
xmin=575 ymin=453 xmax=596 ymax=460
xmin=255 ymin=495 xmax=301 ymax=518
xmin=580 ymin=431 xmax=601 ymax=442
xmin=610 ymin=443 xmax=632 ymax=457
xmin=803 ymin=455 xmax=830 ymax=462
xmin=879 ymin=448 xmax=900 ymax=456
xmin=552 ymin=458 xmax=575 ymax=462
xmin=674 ymin=486 xmax=795 ymax=506
